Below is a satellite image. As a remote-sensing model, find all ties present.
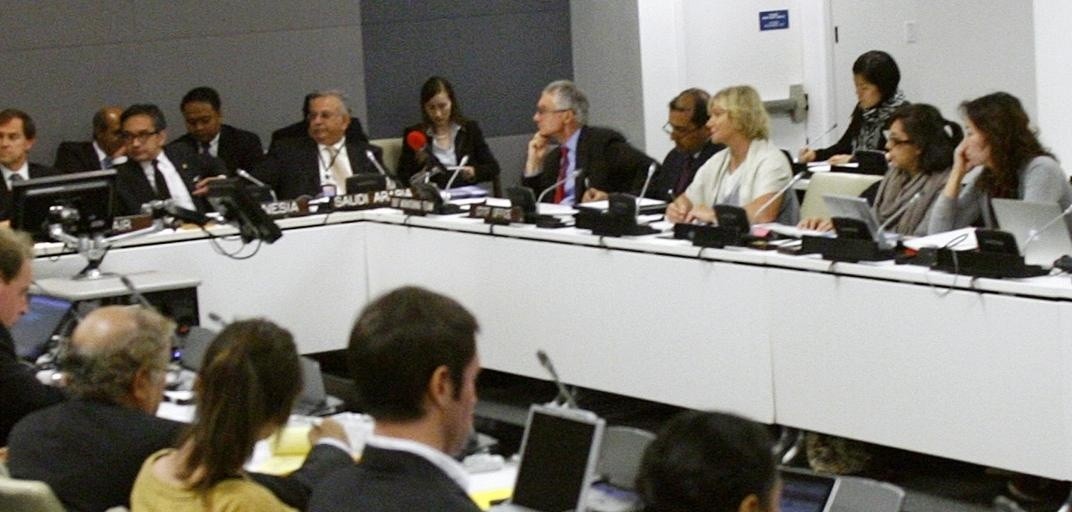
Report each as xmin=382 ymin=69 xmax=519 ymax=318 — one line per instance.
xmin=9 ymin=174 xmax=24 ymax=184
xmin=101 ymin=155 xmax=113 ymax=170
xmin=200 ymin=143 xmax=216 ymax=171
xmin=152 ymin=160 xmax=172 ymax=202
xmin=326 ymin=146 xmax=350 ymax=194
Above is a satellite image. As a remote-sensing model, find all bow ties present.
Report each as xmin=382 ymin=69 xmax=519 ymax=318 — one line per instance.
xmin=674 ymin=155 xmax=694 ymax=197
xmin=552 ymin=146 xmax=570 ymax=205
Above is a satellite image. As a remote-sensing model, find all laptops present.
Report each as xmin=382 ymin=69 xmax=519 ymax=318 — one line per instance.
xmin=989 ymin=196 xmax=1072 ymax=269
xmin=8 ymin=293 xmax=73 ymax=362
xmin=775 ymin=467 xmax=842 ymax=512
xmin=492 ymin=403 xmax=608 ymax=512
xmin=821 ymin=194 xmax=905 ymax=243
xmin=288 ymin=356 xmax=329 ymax=416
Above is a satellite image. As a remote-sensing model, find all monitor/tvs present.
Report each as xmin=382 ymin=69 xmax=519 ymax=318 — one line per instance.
xmin=13 ymin=168 xmax=119 ymax=250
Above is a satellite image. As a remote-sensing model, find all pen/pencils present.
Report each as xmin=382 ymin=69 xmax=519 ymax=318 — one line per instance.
xmin=425 ymin=165 xmax=431 ymax=183
xmin=807 ymin=137 xmax=808 ymax=153
xmin=312 ymin=422 xmax=320 ymax=430
xmin=668 ymin=189 xmax=681 ymax=208
xmin=585 ymin=178 xmax=594 ymax=197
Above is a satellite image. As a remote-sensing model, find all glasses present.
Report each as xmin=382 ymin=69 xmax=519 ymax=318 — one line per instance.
xmin=888 ymin=136 xmax=913 ymax=147
xmin=661 ymin=120 xmax=699 ymax=140
xmin=120 ymin=130 xmax=158 ymax=146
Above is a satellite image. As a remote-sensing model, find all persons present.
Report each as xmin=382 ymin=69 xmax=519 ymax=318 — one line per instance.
xmin=164 ymin=85 xmax=262 ymax=181
xmin=304 ymin=285 xmax=493 ymax=512
xmin=128 ymin=312 xmax=307 ymax=511
xmin=653 ymin=85 xmax=726 ymax=199
xmin=8 ymin=300 xmax=357 ymax=511
xmin=519 ymin=80 xmax=666 ymax=207
xmin=392 ymin=76 xmax=504 ymax=192
xmin=633 ymin=405 xmax=785 ymax=511
xmin=2 ymin=219 xmax=78 ymax=455
xmin=51 ymin=105 xmax=119 ymax=170
xmin=273 ymin=91 xmax=384 ymax=202
xmin=796 ymin=47 xmax=912 ymax=170
xmin=107 ymin=100 xmax=233 ymax=232
xmin=920 ymin=87 xmax=1071 ymax=279
xmin=661 ymin=86 xmax=801 ymax=230
xmin=0 ymin=108 xmax=65 ymax=226
xmin=802 ymin=99 xmax=963 ymax=246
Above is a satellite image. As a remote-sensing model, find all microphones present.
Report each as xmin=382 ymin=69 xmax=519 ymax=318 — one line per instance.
xmin=440 ymin=151 xmax=470 ymax=200
xmin=209 ymin=312 xmax=233 ymax=330
xmin=536 ymin=350 xmax=579 ymax=409
xmin=749 ymin=170 xmax=807 ymax=237
xmin=1019 ymin=203 xmax=1072 ymax=255
xmin=799 ymin=121 xmax=838 ymax=163
xmin=873 ymin=191 xmax=924 ymax=249
xmin=235 ymin=167 xmax=286 ymax=216
xmin=366 ymin=148 xmax=397 ymax=190
xmin=535 ymin=167 xmax=584 ymax=214
xmin=122 ymin=276 xmax=160 ymax=316
xmin=636 ymin=161 xmax=665 ymax=225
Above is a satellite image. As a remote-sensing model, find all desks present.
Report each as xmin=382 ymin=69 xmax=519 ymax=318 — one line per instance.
xmin=2 ymin=123 xmax=1071 ymax=511
